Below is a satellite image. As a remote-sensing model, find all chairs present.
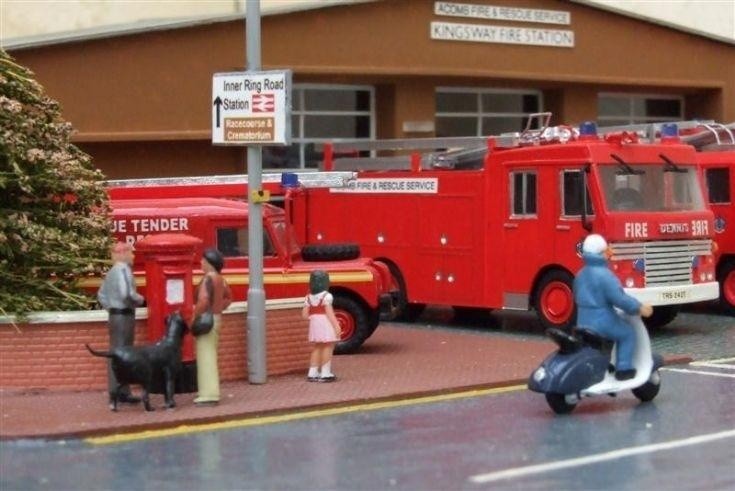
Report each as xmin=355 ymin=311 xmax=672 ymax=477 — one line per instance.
xmin=612 ymin=189 xmax=648 ymax=212
